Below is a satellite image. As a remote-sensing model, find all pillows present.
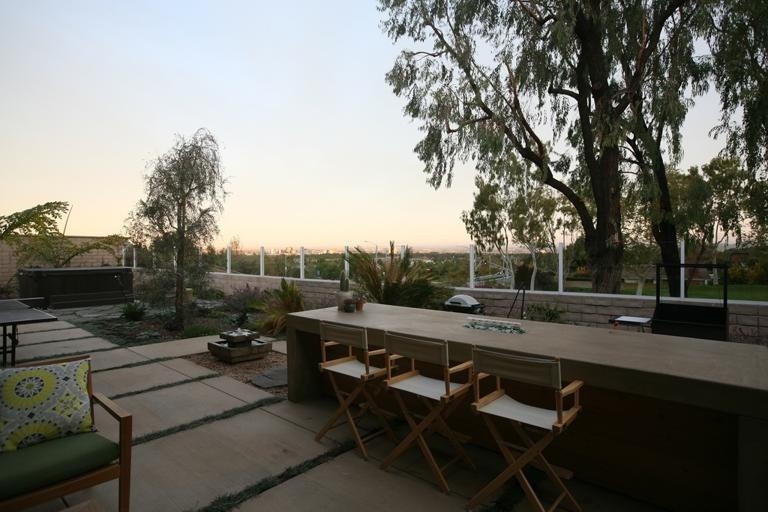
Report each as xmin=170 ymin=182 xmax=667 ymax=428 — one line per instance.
xmin=0 ymin=357 xmax=97 ymax=453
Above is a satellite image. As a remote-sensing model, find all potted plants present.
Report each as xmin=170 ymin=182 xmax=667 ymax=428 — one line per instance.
xmin=337 ymin=271 xmax=365 ymax=314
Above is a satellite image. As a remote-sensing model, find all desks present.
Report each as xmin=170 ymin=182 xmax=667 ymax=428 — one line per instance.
xmin=0 ymin=299 xmax=58 ymax=367
xmin=285 ymin=301 xmax=767 ymax=512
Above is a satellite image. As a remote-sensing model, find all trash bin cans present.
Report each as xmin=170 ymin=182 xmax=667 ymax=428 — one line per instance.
xmin=443 ymin=294 xmax=485 ymax=315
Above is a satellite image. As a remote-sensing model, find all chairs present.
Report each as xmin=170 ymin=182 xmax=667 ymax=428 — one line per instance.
xmin=0 ymin=354 xmax=134 ymax=511
